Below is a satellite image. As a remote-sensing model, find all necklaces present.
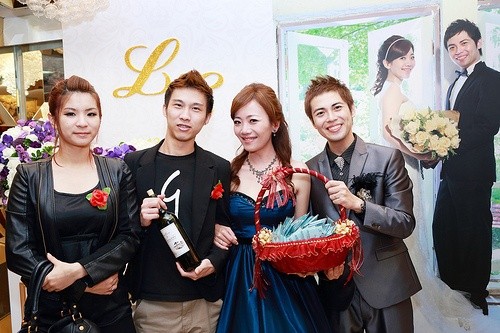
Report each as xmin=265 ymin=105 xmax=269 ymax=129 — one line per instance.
xmin=245 ymin=153 xmax=279 ymax=184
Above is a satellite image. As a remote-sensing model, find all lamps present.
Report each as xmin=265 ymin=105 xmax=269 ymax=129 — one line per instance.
xmin=17 ymin=0 xmax=108 ymax=24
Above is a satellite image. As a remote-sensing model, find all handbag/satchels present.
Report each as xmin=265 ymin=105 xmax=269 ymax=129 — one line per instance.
xmin=17 ymin=259 xmax=102 ymax=333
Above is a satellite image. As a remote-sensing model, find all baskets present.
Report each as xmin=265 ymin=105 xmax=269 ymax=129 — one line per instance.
xmin=253 ymin=168 xmax=353 ymax=277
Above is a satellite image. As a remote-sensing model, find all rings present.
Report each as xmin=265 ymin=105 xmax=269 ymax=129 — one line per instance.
xmin=333 ymin=194 xmax=337 ymax=200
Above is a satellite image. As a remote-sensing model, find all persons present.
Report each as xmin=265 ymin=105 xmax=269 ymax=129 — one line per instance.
xmin=122 ymin=69 xmax=233 ymax=333
xmin=370 ymin=35 xmax=483 ymax=333
xmin=304 ymin=73 xmax=423 ymax=333
xmin=213 ymin=82 xmax=338 ymax=333
xmin=430 ymin=18 xmax=500 ymax=316
xmin=4 ymin=75 xmax=142 ymax=333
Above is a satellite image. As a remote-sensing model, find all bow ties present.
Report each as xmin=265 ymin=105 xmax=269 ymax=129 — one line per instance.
xmin=454 ymin=69 xmax=469 ymax=78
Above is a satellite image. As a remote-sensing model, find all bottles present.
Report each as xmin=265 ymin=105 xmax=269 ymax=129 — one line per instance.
xmin=147 ymin=189 xmax=201 ymax=273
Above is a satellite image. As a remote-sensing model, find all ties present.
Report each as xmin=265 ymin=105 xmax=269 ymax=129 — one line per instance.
xmin=334 ymin=156 xmax=344 ymax=171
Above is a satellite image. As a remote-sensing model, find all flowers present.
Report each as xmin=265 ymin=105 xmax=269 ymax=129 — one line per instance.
xmin=0 ymin=117 xmax=136 ymax=211
xmin=388 ymin=100 xmax=462 ymax=164
xmin=211 ymin=179 xmax=225 ymax=199
